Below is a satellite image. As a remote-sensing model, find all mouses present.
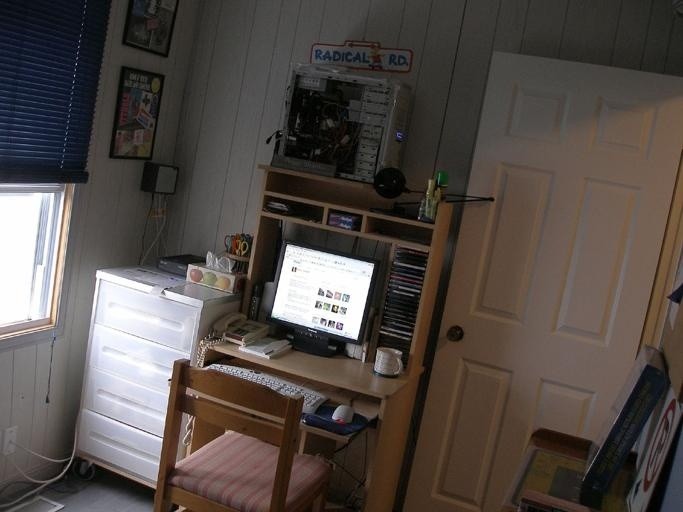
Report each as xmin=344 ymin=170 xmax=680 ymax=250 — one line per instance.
xmin=331 ymin=404 xmax=355 ymax=425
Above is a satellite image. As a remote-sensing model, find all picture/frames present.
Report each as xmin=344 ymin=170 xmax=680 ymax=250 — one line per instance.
xmin=121 ymin=2 xmax=176 ymax=59
xmin=108 ymin=66 xmax=165 ymax=159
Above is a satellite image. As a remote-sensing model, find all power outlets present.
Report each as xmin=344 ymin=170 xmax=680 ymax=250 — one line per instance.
xmin=3 ymin=430 xmax=22 ymax=459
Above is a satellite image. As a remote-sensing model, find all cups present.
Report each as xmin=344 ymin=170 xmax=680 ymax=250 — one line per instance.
xmin=372 ymin=347 xmax=404 ymax=378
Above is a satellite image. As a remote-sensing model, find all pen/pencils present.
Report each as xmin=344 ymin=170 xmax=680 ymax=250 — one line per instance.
xmin=224 ymin=233 xmax=240 ymax=255
xmin=240 ymin=233 xmax=251 ymax=241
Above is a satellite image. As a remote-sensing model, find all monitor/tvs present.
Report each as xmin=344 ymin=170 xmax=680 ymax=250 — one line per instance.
xmin=266 ymin=240 xmax=381 ymax=358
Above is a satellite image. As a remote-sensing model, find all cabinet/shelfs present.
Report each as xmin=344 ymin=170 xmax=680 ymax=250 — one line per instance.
xmin=241 ymin=164 xmax=454 ymax=371
xmin=70 ymin=267 xmax=241 ymax=508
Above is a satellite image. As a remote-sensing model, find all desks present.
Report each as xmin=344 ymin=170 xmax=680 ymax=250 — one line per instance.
xmin=499 ymin=426 xmax=639 ymax=512
xmin=181 ymin=323 xmax=425 ymax=512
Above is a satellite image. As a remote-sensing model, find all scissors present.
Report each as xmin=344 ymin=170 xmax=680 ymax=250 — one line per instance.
xmin=235 ymin=240 xmax=249 ymax=274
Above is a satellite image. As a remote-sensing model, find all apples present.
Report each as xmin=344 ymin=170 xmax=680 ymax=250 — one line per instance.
xmin=189 ymin=268 xmax=230 ymax=290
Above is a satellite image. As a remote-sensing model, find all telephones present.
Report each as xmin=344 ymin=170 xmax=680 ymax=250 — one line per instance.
xmin=211 ymin=310 xmax=271 ymax=350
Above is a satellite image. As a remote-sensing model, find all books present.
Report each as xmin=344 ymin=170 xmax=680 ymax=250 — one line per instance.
xmin=238 ymin=337 xmax=293 ymax=360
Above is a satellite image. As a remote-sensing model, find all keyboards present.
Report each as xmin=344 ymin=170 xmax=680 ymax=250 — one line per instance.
xmin=201 ymin=362 xmax=329 ymax=415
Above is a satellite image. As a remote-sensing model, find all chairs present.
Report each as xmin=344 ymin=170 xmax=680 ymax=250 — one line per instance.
xmin=151 ymin=359 xmax=334 ymax=512
